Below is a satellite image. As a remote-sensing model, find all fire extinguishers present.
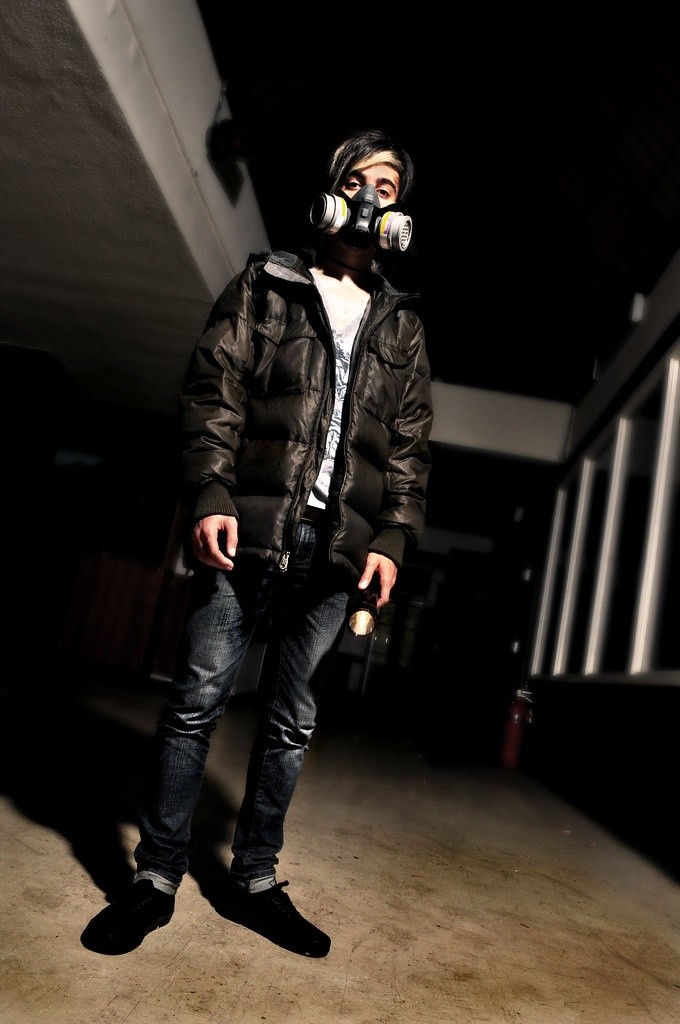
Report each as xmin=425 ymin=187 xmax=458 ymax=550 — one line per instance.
xmin=501 ymin=689 xmax=534 ymax=768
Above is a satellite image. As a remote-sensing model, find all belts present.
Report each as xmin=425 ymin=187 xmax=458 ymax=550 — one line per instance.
xmin=303 ymin=506 xmax=341 ymax=523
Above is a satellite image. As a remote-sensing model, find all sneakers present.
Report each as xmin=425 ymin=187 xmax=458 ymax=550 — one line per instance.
xmin=212 ymin=871 xmax=332 ymax=958
xmin=81 ymin=879 xmax=173 ymax=955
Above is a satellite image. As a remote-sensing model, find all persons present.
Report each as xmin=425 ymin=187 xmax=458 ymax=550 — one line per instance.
xmin=80 ymin=131 xmax=432 ymax=958
